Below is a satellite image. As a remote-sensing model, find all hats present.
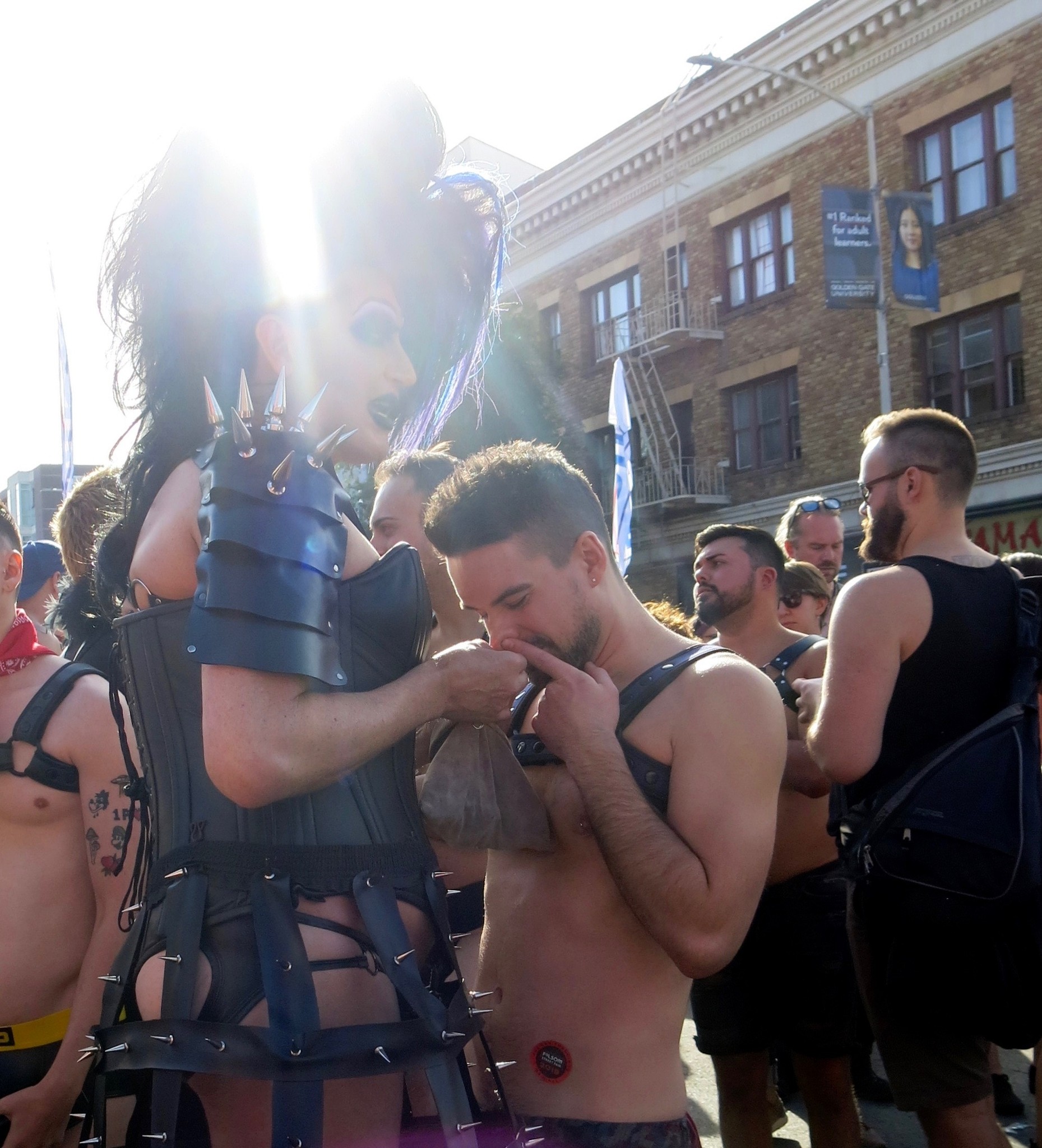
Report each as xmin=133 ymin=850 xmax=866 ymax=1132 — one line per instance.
xmin=15 ymin=540 xmax=66 ymax=601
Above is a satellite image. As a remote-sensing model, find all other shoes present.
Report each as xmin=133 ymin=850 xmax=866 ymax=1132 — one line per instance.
xmin=991 ymin=1073 xmax=1028 ymax=1131
xmin=1027 ymin=1064 xmax=1038 ymax=1095
xmin=850 ymin=1068 xmax=897 ymax=1109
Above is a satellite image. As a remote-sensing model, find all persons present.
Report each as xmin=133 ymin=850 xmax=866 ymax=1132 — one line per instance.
xmin=65 ymin=64 xmax=545 ymax=1146
xmin=423 ymin=439 xmax=788 ymax=1148
xmin=0 ymin=519 xmax=146 ymax=1148
xmin=792 ymin=409 xmax=1042 ymax=1148
xmin=894 ymin=192 xmax=943 ymax=307
xmin=1 ymin=63 xmax=1042 ymax=1148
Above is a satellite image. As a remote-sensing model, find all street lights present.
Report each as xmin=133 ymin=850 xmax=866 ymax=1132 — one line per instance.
xmin=687 ymin=51 xmax=892 ymax=415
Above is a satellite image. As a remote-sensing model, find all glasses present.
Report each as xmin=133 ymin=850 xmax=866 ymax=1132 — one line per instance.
xmin=788 ymin=497 xmax=842 ymax=532
xmin=778 ymin=587 xmax=819 ymax=609
xmin=857 ymin=464 xmax=938 ymax=507
